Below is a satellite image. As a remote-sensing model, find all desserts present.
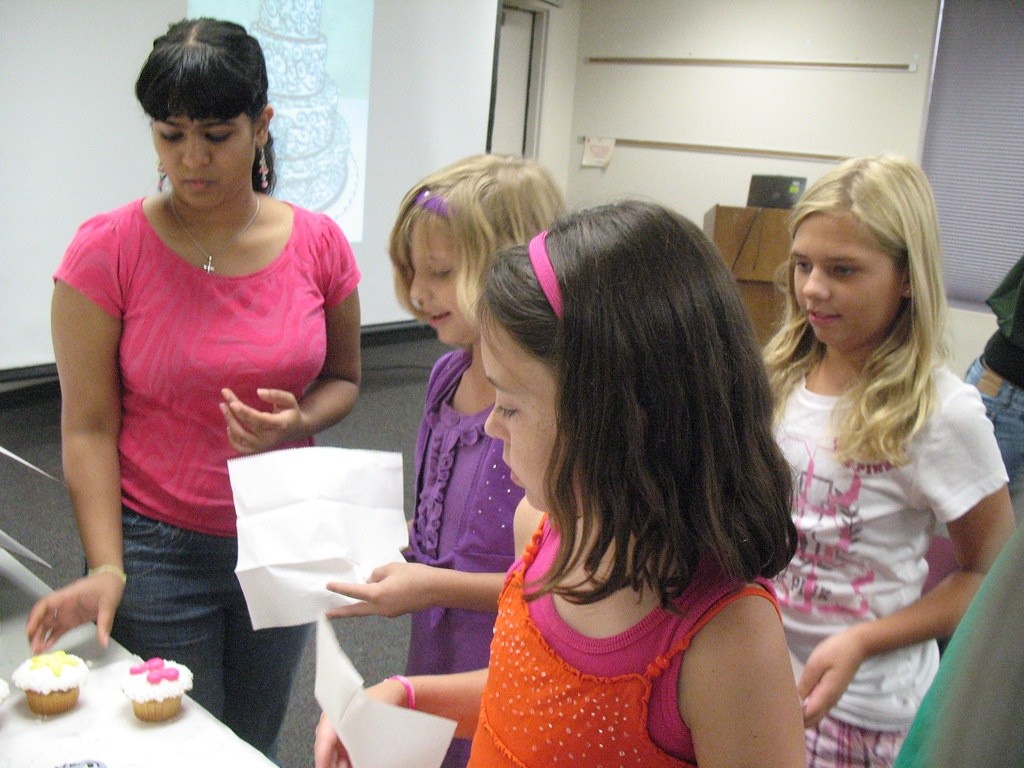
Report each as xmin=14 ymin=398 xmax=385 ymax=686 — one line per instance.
xmin=12 ymin=650 xmax=90 ymax=714
xmin=120 ymin=658 xmax=193 ymax=721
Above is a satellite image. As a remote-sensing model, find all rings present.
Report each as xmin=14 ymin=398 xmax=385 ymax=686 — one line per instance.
xmin=54 ymin=610 xmax=59 ymax=621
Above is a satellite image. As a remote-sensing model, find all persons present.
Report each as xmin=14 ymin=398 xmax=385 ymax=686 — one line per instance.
xmin=313 ymin=201 xmax=808 ymax=768
xmin=323 ymin=156 xmax=570 ymax=768
xmin=26 ymin=18 xmax=363 ymax=759
xmin=761 ymin=156 xmax=1017 ymax=768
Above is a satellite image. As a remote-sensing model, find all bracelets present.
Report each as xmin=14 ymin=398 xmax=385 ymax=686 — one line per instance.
xmin=87 ymin=564 xmax=128 ymax=582
xmin=380 ymin=672 xmax=418 ymax=710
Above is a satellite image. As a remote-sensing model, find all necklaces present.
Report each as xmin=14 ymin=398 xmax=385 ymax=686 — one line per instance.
xmin=166 ymin=193 xmax=261 ymax=279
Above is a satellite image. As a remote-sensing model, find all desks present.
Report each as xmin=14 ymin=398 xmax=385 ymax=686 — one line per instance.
xmin=0 ymin=545 xmax=282 ymax=768
